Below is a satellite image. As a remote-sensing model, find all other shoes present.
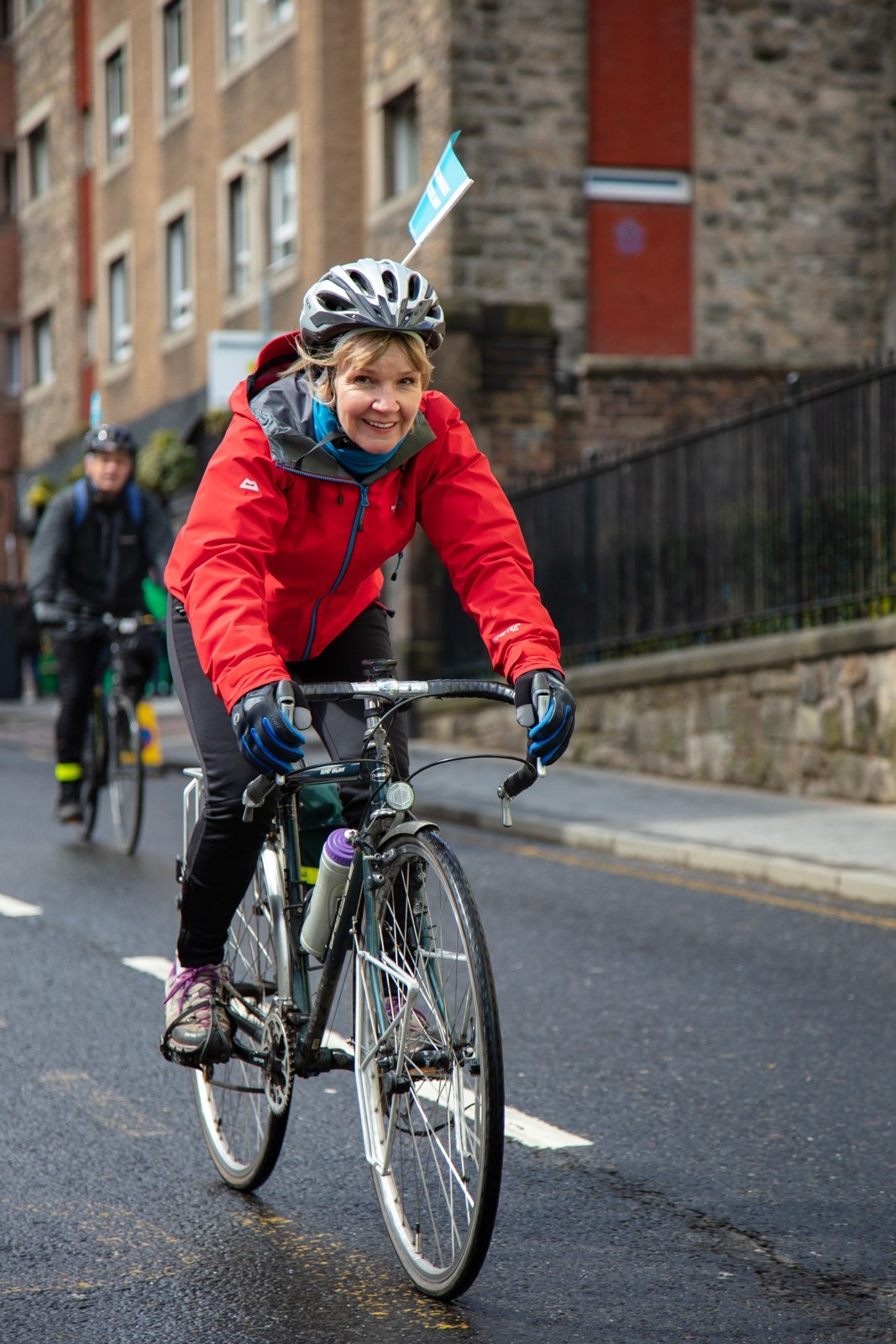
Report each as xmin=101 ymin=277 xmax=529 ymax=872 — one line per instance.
xmin=57 ymin=799 xmax=83 ymax=825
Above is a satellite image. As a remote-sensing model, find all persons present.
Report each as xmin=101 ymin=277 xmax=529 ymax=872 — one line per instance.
xmin=27 ymin=426 xmax=175 ymax=827
xmin=161 ymin=258 xmax=575 ymax=1064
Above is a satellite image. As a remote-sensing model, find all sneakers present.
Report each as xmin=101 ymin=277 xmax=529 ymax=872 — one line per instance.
xmin=387 ymin=993 xmax=440 ymax=1059
xmin=163 ymin=952 xmax=235 ymax=1064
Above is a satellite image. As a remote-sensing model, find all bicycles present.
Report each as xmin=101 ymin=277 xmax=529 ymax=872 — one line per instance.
xmin=37 ymin=602 xmax=164 ymax=857
xmin=161 ymin=658 xmax=550 ymax=1304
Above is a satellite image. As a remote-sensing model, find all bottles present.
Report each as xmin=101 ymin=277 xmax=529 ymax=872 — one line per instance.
xmin=298 ymin=827 xmax=360 ymax=960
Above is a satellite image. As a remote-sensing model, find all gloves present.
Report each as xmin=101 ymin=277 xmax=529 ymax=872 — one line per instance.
xmin=228 ymin=679 xmax=313 ymax=779
xmin=31 ymin=600 xmax=71 ymax=629
xmin=514 ymin=665 xmax=578 ymax=770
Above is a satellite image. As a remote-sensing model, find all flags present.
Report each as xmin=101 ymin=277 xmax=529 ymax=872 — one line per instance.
xmin=408 ymin=130 xmax=469 ymax=243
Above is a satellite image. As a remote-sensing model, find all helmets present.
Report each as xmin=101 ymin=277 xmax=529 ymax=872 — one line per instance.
xmin=81 ymin=421 xmax=138 ymax=457
xmin=300 ymin=259 xmax=444 ymax=352
